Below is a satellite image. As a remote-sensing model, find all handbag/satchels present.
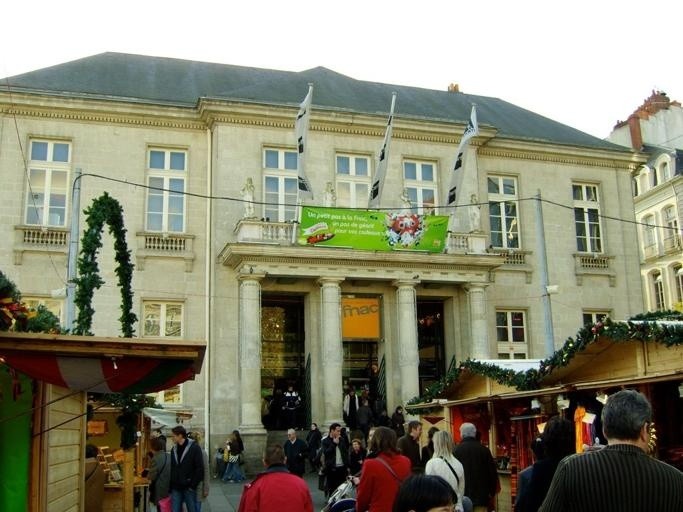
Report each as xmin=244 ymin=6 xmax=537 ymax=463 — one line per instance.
xmin=228 ymin=455 xmax=239 ymax=463
xmin=158 ymin=497 xmax=172 ymax=512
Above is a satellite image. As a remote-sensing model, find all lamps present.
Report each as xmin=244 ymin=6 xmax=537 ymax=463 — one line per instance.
xmin=557 ymin=394 xmax=570 ymax=410
xmin=595 ymin=390 xmax=609 ymax=404
xmin=531 ymin=398 xmax=543 ymax=410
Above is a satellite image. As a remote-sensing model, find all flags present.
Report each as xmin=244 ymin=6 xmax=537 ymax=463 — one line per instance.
xmin=368 ymin=95 xmax=396 ymax=208
xmin=445 ymin=106 xmax=480 ymax=214
xmin=294 ymin=87 xmax=314 ymax=200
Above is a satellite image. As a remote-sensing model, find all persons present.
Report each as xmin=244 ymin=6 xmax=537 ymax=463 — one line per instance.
xmin=537 ymin=389 xmax=681 ymax=511
xmin=187 ymin=431 xmax=209 ymax=511
xmin=515 ymin=434 xmax=546 ymax=505
xmin=84 ymin=444 xmax=106 ymax=511
xmin=172 ymin=425 xmax=202 ymax=511
xmin=148 ymin=439 xmax=173 ymax=512
xmin=218 ymin=378 xmax=502 ymax=511
xmin=514 ymin=415 xmax=576 ymax=512
xmin=141 ymin=428 xmax=167 ymax=478
